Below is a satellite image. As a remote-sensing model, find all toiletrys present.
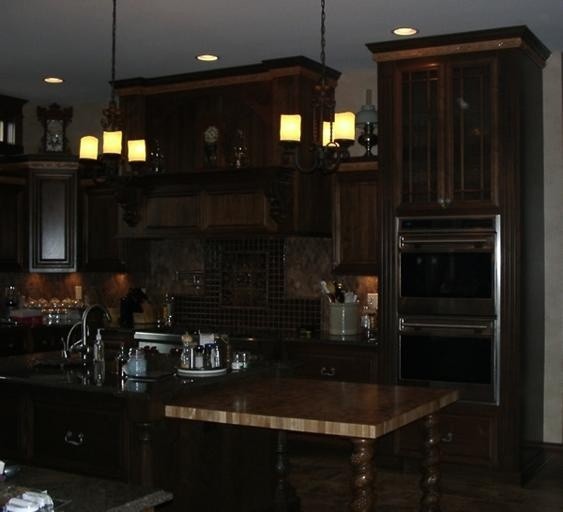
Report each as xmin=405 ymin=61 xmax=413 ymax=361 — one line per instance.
xmin=93 ymin=328 xmax=105 ymax=362
xmin=93 ymin=361 xmax=106 ymax=388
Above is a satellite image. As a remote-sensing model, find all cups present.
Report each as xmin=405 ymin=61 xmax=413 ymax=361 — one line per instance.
xmin=360 ymin=312 xmax=376 ymax=335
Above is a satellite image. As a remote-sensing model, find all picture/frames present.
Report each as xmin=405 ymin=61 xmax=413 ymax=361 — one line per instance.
xmin=216 ymin=233 xmax=332 ymax=312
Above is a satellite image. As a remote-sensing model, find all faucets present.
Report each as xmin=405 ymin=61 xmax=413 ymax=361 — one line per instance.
xmin=60 ymin=320 xmax=90 ymax=352
xmin=81 ymin=303 xmax=111 ymax=349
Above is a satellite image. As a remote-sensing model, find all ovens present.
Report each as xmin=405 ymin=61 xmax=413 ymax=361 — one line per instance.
xmin=378 ymin=210 xmax=501 ymax=407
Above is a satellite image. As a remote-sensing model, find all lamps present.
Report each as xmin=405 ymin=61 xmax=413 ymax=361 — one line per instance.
xmin=277 ymin=1 xmax=377 ymax=174
xmin=76 ymin=1 xmax=163 ymax=184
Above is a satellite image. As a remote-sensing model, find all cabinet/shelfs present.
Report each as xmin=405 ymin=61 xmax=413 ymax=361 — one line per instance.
xmin=377 ymin=46 xmax=520 ymax=212
xmin=398 ymin=412 xmax=503 ymax=467
xmin=329 ymin=173 xmax=382 ymax=276
xmin=1 ymin=159 xmax=124 ymax=272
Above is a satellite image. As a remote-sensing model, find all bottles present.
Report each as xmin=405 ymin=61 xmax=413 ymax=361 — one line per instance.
xmin=150 ymin=144 xmax=169 ymax=174
xmin=320 ymin=280 xmax=344 ymax=303
xmin=115 ymin=335 xmax=250 ymax=380
xmin=2 ymin=282 xmax=83 ymax=320
xmin=228 ymin=130 xmax=250 ymax=170
xmin=156 ymin=293 xmax=176 ymax=328
xmin=124 ymin=380 xmax=148 ymax=394
